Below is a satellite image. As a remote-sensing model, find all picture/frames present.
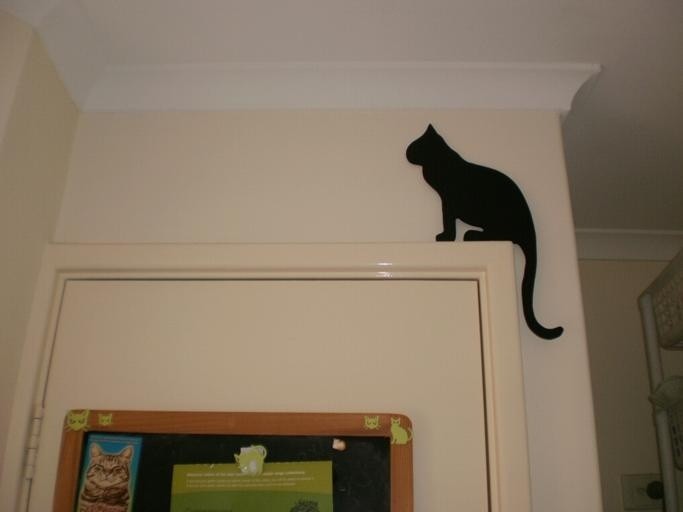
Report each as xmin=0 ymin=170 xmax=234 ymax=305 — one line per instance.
xmin=50 ymin=409 xmax=414 ymax=512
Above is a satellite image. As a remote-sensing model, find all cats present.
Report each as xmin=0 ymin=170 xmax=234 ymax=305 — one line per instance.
xmin=77 ymin=442 xmax=133 ymax=512
